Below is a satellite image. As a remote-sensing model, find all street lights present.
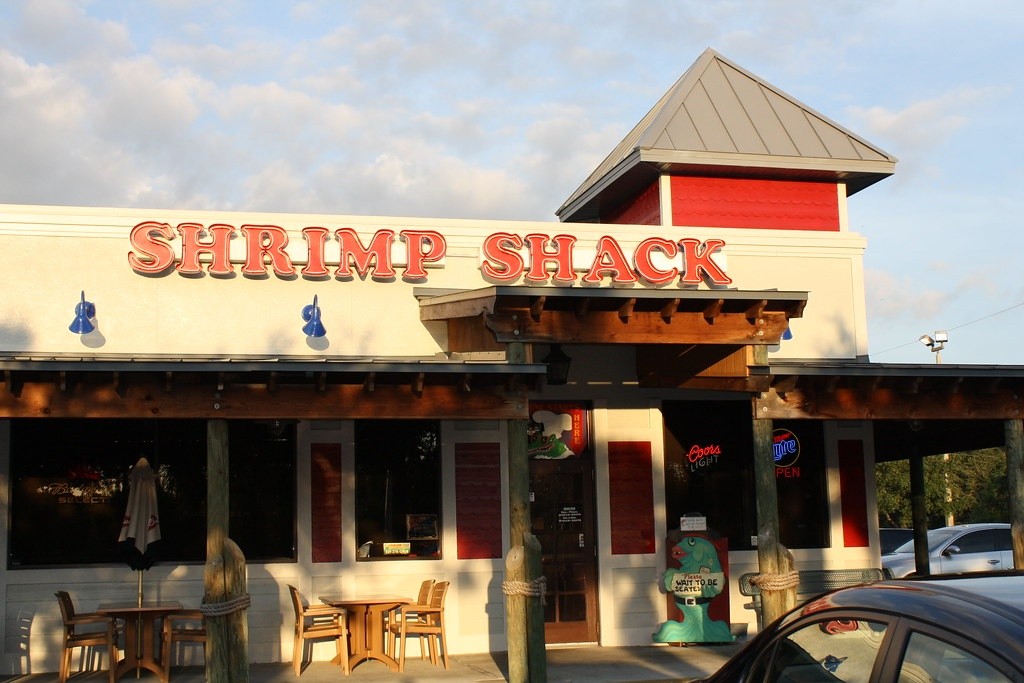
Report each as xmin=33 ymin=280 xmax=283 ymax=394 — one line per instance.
xmin=919 ymin=331 xmax=955 ymax=527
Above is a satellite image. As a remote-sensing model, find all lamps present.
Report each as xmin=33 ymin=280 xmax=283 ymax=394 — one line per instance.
xmin=301 ymin=295 xmax=326 ymax=337
xmin=69 ymin=291 xmax=97 ymax=335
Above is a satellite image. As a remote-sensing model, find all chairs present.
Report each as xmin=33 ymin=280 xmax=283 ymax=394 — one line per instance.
xmin=54 ymin=590 xmax=119 ymax=683
xmin=387 ymin=579 xmax=436 ymax=669
xmin=159 ymin=608 xmax=208 ymax=683
xmin=390 ymin=580 xmax=450 ymax=671
xmin=287 ymin=583 xmax=351 ymax=677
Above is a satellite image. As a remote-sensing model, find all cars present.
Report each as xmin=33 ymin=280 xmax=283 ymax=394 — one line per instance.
xmin=879 ymin=527 xmax=934 ymax=556
xmin=688 ymin=576 xmax=1024 ymax=683
xmin=880 ymin=523 xmax=1015 ymax=581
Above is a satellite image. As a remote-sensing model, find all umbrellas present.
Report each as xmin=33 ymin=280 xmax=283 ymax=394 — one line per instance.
xmin=117 ymin=458 xmax=161 ymax=679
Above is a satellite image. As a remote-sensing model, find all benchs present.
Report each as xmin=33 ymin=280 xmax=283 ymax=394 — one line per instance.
xmin=739 ymin=567 xmax=880 ymax=639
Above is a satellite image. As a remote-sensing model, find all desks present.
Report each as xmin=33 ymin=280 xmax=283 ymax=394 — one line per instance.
xmin=95 ymin=600 xmax=185 ymax=683
xmin=319 ymin=591 xmax=413 ymax=676
